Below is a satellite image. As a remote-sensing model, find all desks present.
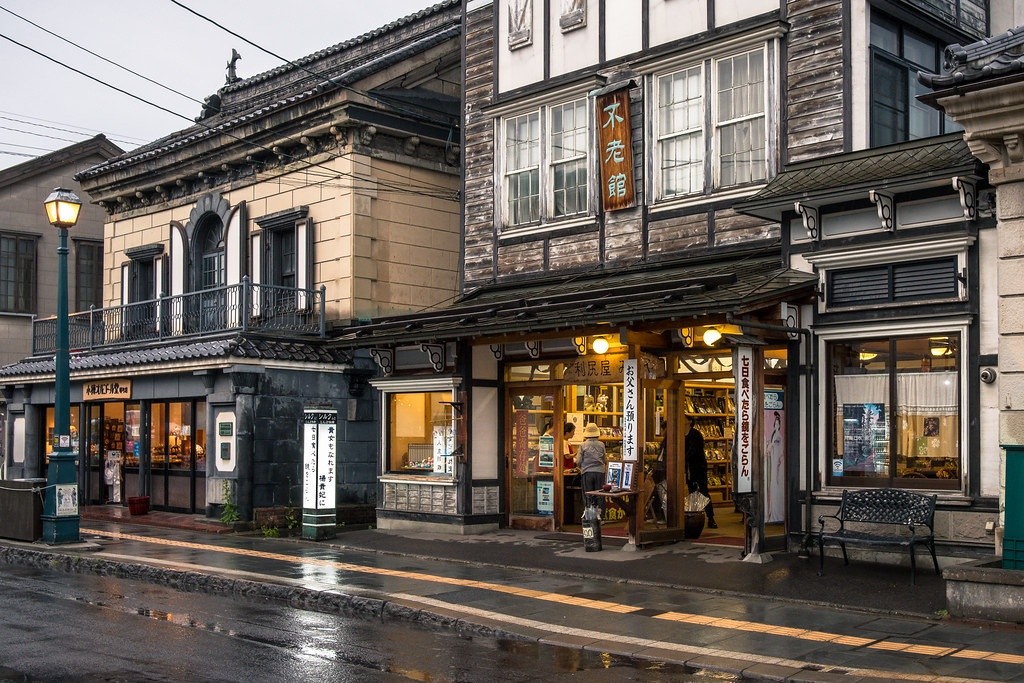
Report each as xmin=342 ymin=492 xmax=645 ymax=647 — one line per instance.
xmin=901 ymin=466 xmax=957 ymax=475
xmin=585 ymin=490 xmax=636 ymax=516
xmin=401 ymin=467 xmax=433 ymax=472
xmin=531 ymin=471 xmax=582 ymax=513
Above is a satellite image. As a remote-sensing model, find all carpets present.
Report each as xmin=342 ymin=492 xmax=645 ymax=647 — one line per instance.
xmin=693 ymin=537 xmax=744 ymax=547
xmin=534 ymin=533 xmax=627 ymax=546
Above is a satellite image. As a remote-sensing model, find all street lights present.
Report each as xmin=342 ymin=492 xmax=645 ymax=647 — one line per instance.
xmin=44 ymin=186 xmax=83 ymax=546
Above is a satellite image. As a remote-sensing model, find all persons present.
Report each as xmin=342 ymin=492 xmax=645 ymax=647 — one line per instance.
xmin=563 ymin=422 xmax=577 ymax=469
xmin=533 ymin=418 xmax=555 ymax=473
xmin=576 ymin=423 xmax=607 ymax=525
xmin=656 ymin=420 xmax=667 ymax=482
xmin=684 ymin=414 xmax=718 ymax=528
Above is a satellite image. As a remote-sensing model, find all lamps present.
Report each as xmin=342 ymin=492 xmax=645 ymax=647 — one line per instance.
xmin=593 ymin=336 xmax=610 ymax=354
xmin=702 ymin=326 xmax=721 ymax=346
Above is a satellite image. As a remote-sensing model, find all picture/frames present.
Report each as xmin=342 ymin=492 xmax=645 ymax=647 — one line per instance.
xmin=605 ymin=459 xmax=636 ymax=491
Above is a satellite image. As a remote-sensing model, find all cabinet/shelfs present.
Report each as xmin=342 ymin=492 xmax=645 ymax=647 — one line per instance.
xmin=644 ymin=386 xmax=735 ymax=507
xmin=512 ymin=383 xmax=621 ymax=468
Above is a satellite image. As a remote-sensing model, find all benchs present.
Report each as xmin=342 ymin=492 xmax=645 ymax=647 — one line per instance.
xmin=817 ymin=489 xmax=941 ymax=587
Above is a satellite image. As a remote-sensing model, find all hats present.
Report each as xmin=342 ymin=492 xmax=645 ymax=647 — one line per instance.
xmin=584 ymin=423 xmax=600 ymax=437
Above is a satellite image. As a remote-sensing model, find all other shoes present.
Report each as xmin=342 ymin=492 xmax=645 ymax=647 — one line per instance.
xmin=708 ymin=518 xmax=718 ymax=529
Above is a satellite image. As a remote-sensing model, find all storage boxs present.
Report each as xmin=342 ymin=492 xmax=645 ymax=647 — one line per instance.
xmin=709 ymin=491 xmax=722 ymax=503
